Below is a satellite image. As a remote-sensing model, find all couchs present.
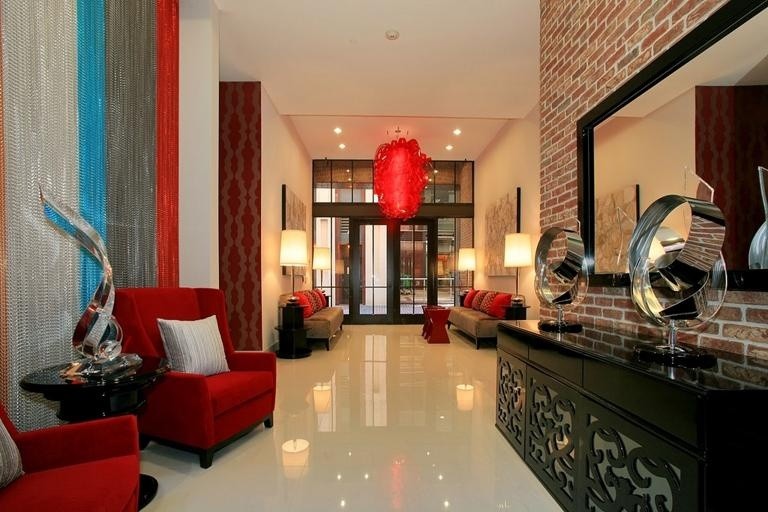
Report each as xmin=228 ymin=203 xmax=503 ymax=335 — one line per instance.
xmin=446 ymin=287 xmax=525 ymax=350
xmin=278 ymin=288 xmax=345 ymax=351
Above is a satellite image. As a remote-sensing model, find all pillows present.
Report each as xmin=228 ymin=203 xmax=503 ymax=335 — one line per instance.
xmin=463 ymin=287 xmax=512 ymax=319
xmin=293 ymin=286 xmax=327 ymax=318
xmin=0 ymin=414 xmax=29 ymax=485
xmin=154 ymin=312 xmax=229 ymax=378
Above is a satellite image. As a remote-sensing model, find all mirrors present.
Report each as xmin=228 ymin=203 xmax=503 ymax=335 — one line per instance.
xmin=572 ymin=0 xmax=768 ymax=296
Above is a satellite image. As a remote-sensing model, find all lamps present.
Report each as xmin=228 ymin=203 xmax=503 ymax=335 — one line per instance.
xmin=312 ymin=246 xmax=333 ymax=293
xmin=279 ymin=227 xmax=312 ymax=304
xmin=458 ymin=248 xmax=478 ymax=293
xmin=503 ymin=231 xmax=534 ymax=307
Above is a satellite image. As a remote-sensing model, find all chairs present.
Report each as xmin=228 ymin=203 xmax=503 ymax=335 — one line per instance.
xmin=103 ymin=282 xmax=281 ymax=470
xmin=1 ymin=376 xmax=147 ymax=510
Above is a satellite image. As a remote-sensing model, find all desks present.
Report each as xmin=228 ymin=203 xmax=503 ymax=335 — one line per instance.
xmin=420 ymin=304 xmax=452 ymax=344
xmin=504 ymin=305 xmax=532 ymax=320
xmin=458 ymin=293 xmax=467 ymax=306
xmin=325 ymin=294 xmax=332 ymax=305
xmin=21 ymin=353 xmax=174 ymax=512
xmin=274 ymin=302 xmax=314 ymax=360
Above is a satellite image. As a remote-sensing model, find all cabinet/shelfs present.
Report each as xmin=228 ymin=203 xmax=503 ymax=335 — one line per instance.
xmin=490 ymin=319 xmax=767 ymax=512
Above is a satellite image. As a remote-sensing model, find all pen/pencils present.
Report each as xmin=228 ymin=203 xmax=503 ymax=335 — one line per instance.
xmin=60 ymin=361 xmax=83 ymax=379
